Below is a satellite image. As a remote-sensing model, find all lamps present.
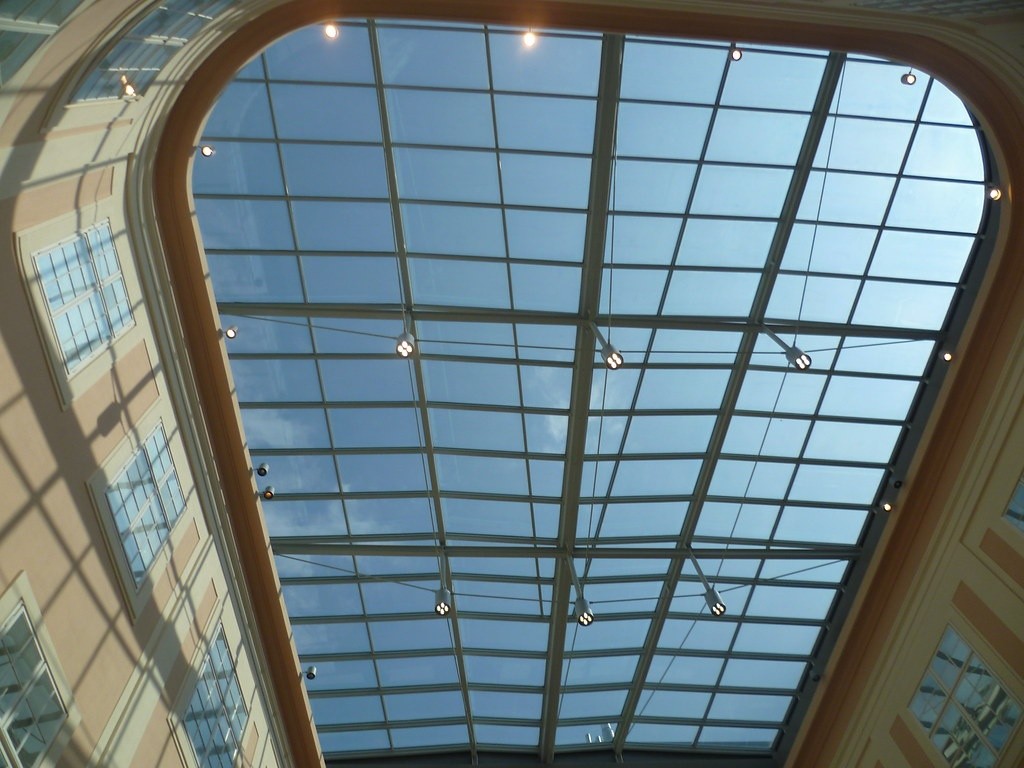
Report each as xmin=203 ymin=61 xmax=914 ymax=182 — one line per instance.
xmin=588 ymin=321 xmax=623 ymax=371
xmin=730 ymin=42 xmax=742 ymax=61
xmin=757 ymin=321 xmax=811 ymax=371
xmin=254 ymin=463 xmax=269 ymax=476
xmin=259 ymin=486 xmax=274 ymax=499
xmin=222 ymin=325 xmax=239 ymax=339
xmin=989 ymin=188 xmax=1001 ymax=200
xmin=808 ymin=669 xmax=822 ymax=682
xmin=324 ymin=19 xmax=337 ymax=38
xmin=522 ymin=27 xmax=535 ymax=46
xmin=302 ymin=666 xmax=317 ymax=679
xmin=198 ymin=144 xmax=215 ymax=156
xmin=566 ymin=552 xmax=594 ymax=627
xmin=901 ymin=68 xmax=916 ymax=85
xmin=395 ymin=308 xmax=414 ymax=359
xmin=686 ymin=546 xmax=727 ymax=616
xmin=943 ymin=353 xmax=951 ymax=360
xmin=883 ymin=503 xmax=892 ymax=512
xmin=435 ymin=553 xmax=451 ymax=616
xmin=888 ymin=475 xmax=902 ymax=489
